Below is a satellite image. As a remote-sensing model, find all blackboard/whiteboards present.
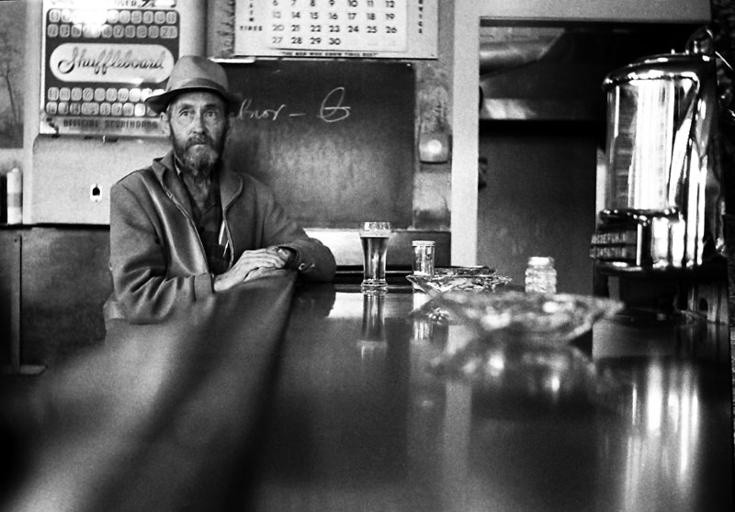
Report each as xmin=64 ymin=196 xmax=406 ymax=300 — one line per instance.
xmin=214 ymin=54 xmax=417 ymax=231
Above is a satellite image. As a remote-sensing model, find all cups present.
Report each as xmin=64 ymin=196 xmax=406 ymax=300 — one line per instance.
xmin=359 ymin=220 xmax=392 ymax=297
xmin=412 ymin=240 xmax=436 ymax=293
xmin=358 ymin=294 xmax=386 ymax=348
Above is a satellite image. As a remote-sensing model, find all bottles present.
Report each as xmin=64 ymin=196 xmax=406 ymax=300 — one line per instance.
xmin=523 ymin=256 xmax=558 ymax=295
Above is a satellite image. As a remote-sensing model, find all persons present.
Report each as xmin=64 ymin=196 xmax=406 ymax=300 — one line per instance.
xmin=109 ymin=56 xmax=335 ymax=325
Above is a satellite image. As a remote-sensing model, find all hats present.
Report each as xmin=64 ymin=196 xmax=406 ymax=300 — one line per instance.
xmin=142 ymin=54 xmax=241 ymax=115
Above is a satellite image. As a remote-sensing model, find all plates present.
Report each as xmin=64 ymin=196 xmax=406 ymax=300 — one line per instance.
xmin=405 ymin=275 xmax=625 ymax=352
